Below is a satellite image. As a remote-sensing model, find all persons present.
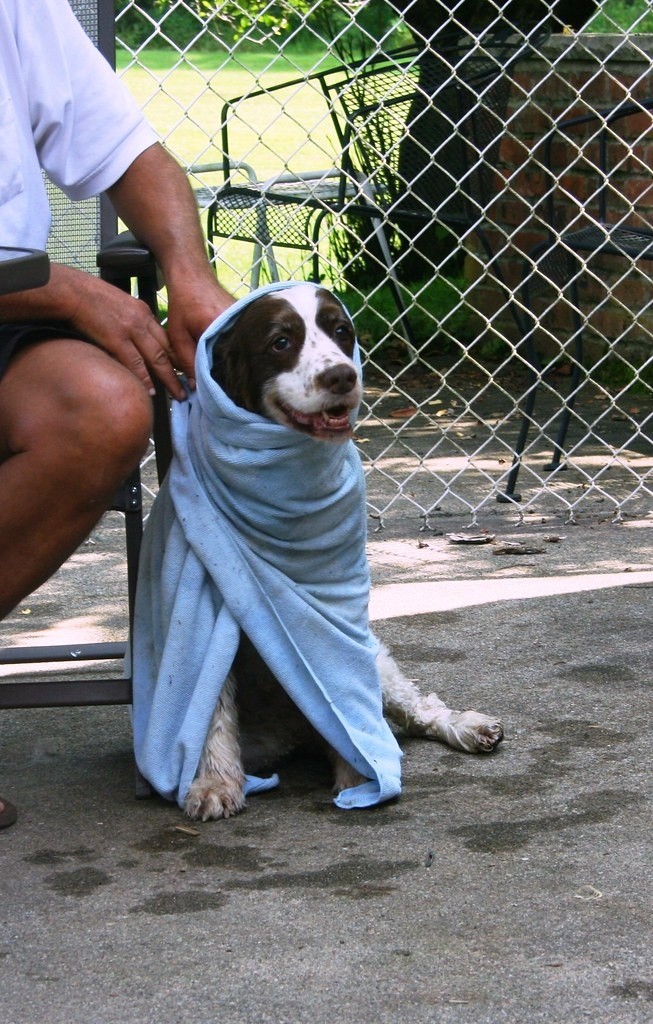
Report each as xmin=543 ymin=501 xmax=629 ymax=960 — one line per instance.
xmin=0 ymin=0 xmax=243 ymax=831
xmin=388 ymin=0 xmax=599 ymax=285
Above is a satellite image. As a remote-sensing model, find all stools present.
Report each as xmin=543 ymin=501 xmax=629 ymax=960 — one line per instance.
xmin=180 ymin=159 xmax=419 ymax=367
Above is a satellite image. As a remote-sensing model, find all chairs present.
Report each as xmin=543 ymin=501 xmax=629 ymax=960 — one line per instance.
xmin=207 ymin=20 xmax=558 ymax=390
xmin=0 ymin=228 xmax=175 ymax=801
xmin=495 ymin=97 xmax=653 ymax=504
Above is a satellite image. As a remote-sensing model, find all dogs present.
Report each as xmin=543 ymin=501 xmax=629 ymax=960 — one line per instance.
xmin=172 ymin=283 xmax=511 ymax=830
